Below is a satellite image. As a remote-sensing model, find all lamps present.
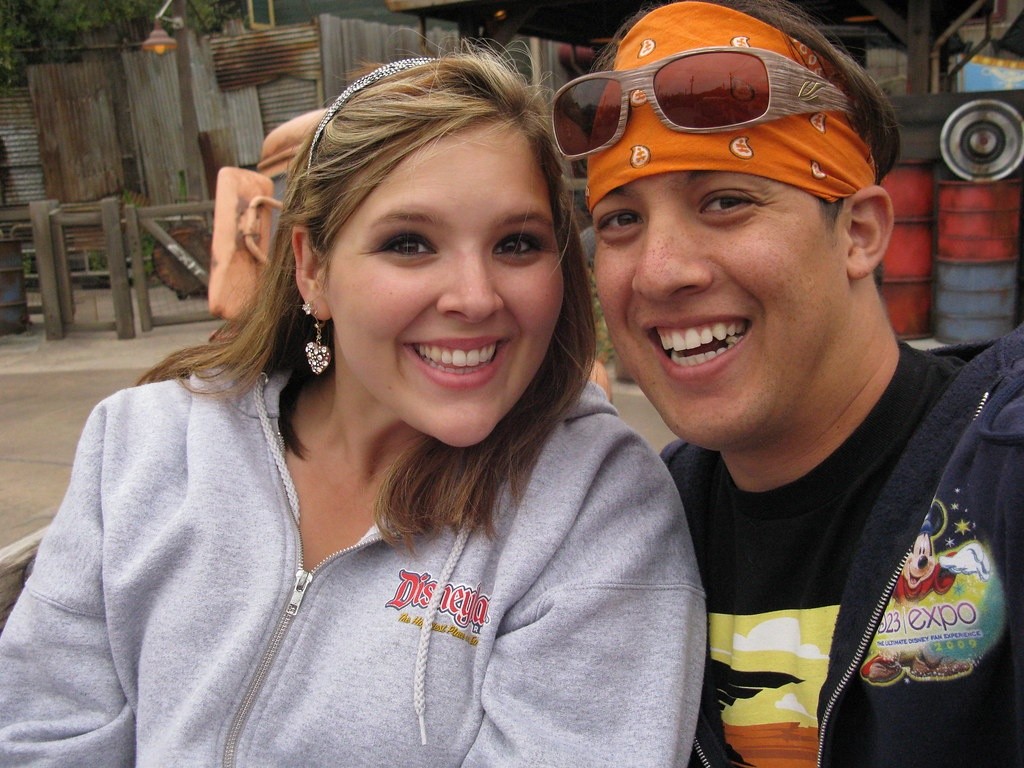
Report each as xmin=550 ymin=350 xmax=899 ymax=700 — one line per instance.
xmin=143 ymin=0 xmax=182 ymax=56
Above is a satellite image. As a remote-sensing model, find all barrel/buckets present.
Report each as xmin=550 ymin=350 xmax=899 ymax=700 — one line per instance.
xmin=0 ymin=238 xmax=30 ymax=336
xmin=876 ymin=158 xmax=1023 ymax=348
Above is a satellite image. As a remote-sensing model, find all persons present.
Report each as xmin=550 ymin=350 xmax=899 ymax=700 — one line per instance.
xmin=0 ymin=48 xmax=708 ymax=768
xmin=550 ymin=0 xmax=1024 ymax=768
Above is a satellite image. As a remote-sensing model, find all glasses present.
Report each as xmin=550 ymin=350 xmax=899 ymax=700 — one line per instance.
xmin=552 ymin=46 xmax=862 ymax=163
